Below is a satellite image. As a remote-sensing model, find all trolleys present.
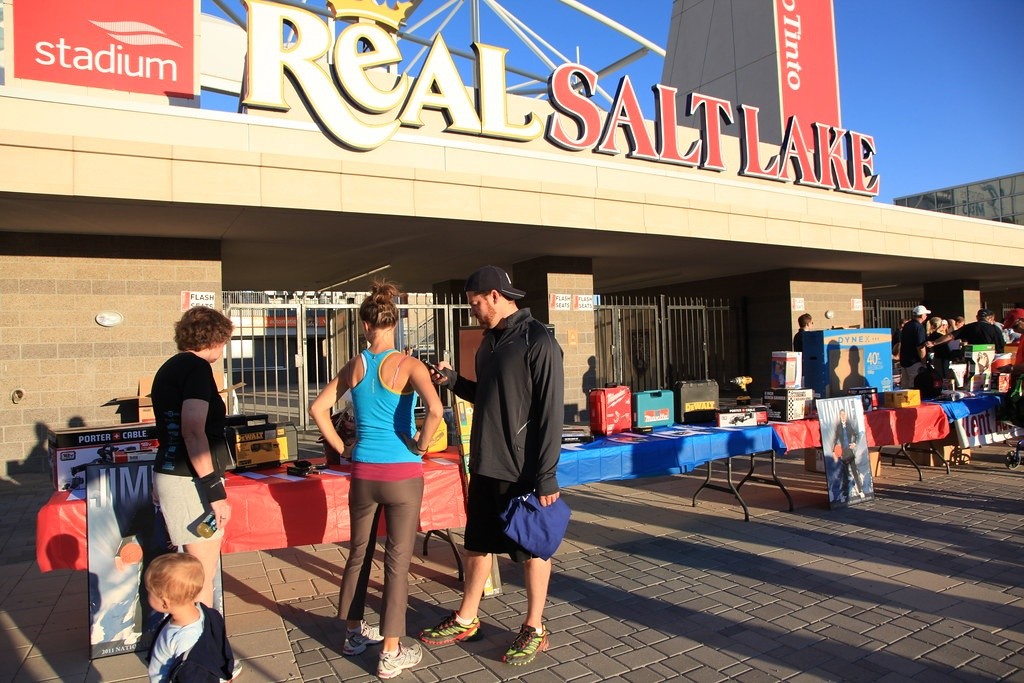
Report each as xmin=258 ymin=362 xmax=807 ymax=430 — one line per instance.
xmin=997 ymin=368 xmax=1024 ymax=471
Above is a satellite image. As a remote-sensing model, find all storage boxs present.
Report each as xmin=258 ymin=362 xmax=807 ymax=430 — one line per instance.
xmin=51 ymin=321 xmax=1018 ymax=493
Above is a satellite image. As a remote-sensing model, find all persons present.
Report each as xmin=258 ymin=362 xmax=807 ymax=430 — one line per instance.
xmin=308 ymin=280 xmax=443 ymax=679
xmin=152 ymin=305 xmax=242 ymax=683
xmin=832 ymin=409 xmax=865 ymax=503
xmin=892 ymin=305 xmax=1024 ymax=451
xmin=794 ymin=313 xmax=813 ymax=376
xmin=144 ymin=553 xmax=234 ymax=683
xmin=419 ymin=265 xmax=564 ymax=665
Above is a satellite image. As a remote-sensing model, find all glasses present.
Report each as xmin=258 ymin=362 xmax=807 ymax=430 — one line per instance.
xmin=1012 ymin=324 xmax=1019 ymax=330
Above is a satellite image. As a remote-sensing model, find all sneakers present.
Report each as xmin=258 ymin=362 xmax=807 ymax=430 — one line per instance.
xmin=343 ymin=619 xmax=384 ymax=655
xmin=502 ymin=624 xmax=550 ymax=666
xmin=377 ymin=642 xmax=423 ymax=679
xmin=418 ymin=610 xmax=482 ymax=646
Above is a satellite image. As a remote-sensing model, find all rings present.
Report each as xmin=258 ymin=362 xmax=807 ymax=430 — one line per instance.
xmin=221 ymin=516 xmax=226 ymax=519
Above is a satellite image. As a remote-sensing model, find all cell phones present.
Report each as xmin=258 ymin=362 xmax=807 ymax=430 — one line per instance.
xmin=422 ymin=358 xmax=445 ymax=378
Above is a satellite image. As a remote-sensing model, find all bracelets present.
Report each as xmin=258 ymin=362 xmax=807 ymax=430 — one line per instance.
xmin=199 ymin=471 xmax=227 ymax=503
xmin=416 ymin=443 xmax=428 ymax=452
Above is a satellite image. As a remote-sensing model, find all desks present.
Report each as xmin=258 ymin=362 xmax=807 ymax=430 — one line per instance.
xmin=33 ymin=390 xmax=1023 ymax=580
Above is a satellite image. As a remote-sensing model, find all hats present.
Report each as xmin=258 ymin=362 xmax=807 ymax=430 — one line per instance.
xmin=1001 ymin=309 xmax=1024 ymax=329
xmin=464 ymin=265 xmax=526 ymax=299
xmin=912 ymin=305 xmax=931 ymax=315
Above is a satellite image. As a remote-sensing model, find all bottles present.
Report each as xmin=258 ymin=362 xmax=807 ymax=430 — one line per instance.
xmin=197 ymin=512 xmax=220 ymax=538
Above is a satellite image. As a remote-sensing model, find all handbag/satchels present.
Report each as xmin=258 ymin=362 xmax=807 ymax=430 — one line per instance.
xmin=500 ymin=493 xmax=572 ymax=561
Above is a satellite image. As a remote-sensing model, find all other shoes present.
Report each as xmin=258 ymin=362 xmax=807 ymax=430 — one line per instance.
xmin=219 ymin=660 xmax=243 ymax=683
